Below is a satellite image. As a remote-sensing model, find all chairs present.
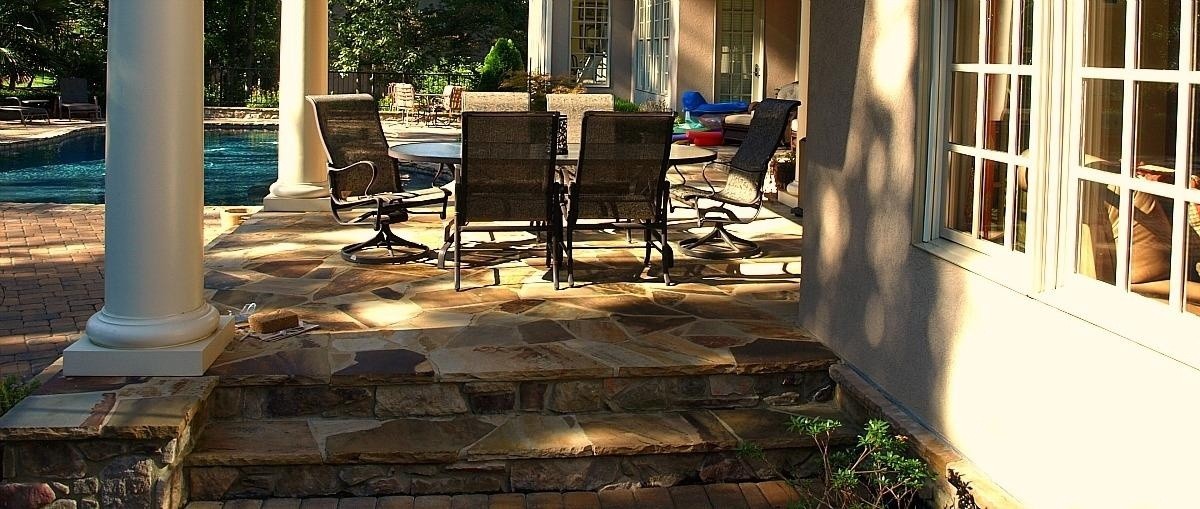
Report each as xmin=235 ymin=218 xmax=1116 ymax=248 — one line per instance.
xmin=556 ymin=109 xmax=674 ymax=287
xmin=304 ymin=93 xmax=454 ymax=263
xmin=59 ymin=78 xmax=102 ymax=122
xmin=667 ymin=97 xmax=800 ymax=261
xmin=545 ymin=93 xmax=633 ymax=243
xmin=681 ymin=82 xmax=804 ymax=148
xmin=387 ymin=82 xmax=463 ymax=127
xmin=452 ymin=109 xmax=562 ymax=290
xmin=460 ymin=91 xmax=530 ymax=141
xmin=1 ymin=96 xmax=51 ymax=126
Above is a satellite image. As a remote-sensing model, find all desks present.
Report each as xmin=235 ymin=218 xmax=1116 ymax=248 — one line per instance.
xmin=387 ymin=141 xmax=717 ymax=268
xmin=22 ymin=99 xmax=50 ymax=125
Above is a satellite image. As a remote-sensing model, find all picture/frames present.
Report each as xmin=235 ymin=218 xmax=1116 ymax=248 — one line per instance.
xmin=579 ymin=24 xmax=601 ymax=50
xmin=578 ymin=2 xmax=608 ymax=20
